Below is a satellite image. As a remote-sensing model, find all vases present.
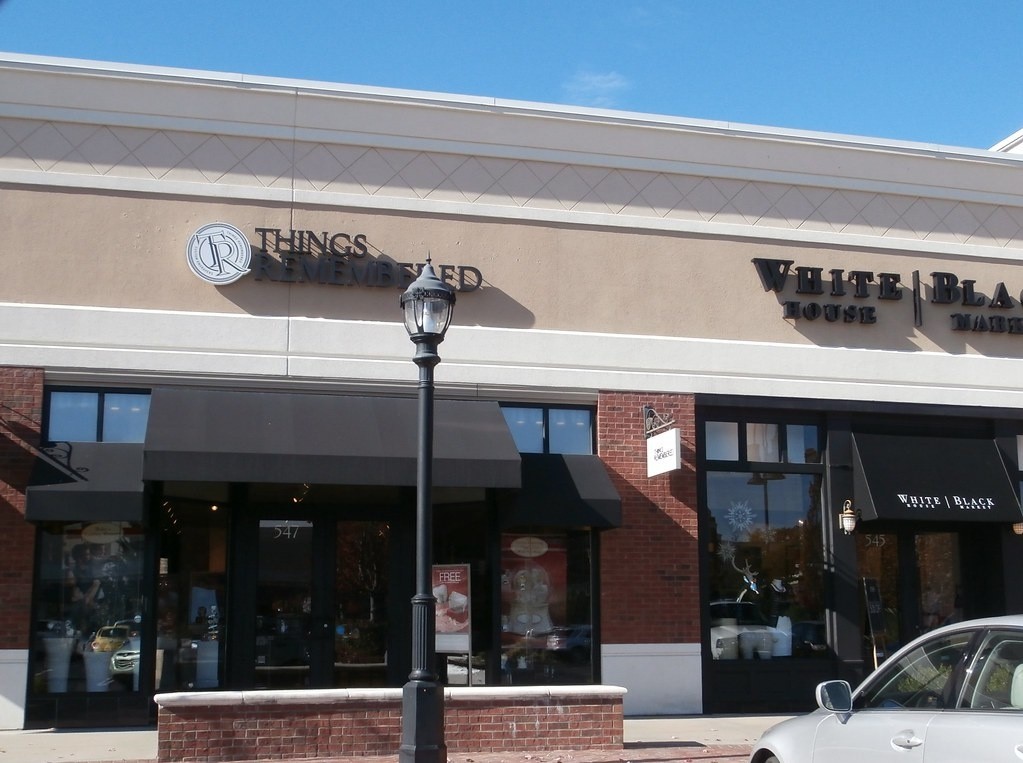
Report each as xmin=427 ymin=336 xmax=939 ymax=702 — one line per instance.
xmin=42 ymin=638 xmax=78 ymax=693
xmin=83 ymin=652 xmax=114 ymax=691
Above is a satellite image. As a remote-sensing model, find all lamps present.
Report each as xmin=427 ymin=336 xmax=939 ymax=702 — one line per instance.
xmin=838 ymin=499 xmax=857 ymax=535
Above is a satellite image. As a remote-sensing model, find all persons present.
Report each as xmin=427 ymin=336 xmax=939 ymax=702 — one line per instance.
xmin=196 ymin=607 xmax=210 ymax=623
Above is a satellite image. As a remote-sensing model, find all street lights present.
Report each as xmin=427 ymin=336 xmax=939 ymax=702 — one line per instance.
xmin=399 ymin=249 xmax=457 ymax=763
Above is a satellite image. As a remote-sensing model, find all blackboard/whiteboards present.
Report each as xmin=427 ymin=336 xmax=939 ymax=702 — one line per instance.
xmin=862 ymin=576 xmax=888 ymax=637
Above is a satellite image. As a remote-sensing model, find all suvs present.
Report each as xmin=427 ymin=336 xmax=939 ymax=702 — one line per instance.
xmin=710 ymin=601 xmax=791 ymax=662
xmin=750 ymin=614 xmax=1023 ymax=763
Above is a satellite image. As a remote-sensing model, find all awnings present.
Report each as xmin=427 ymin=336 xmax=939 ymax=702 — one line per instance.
xmin=142 ymin=388 xmax=522 ymax=488
xmin=482 ymin=453 xmax=622 ymax=532
xmin=27 ymin=445 xmax=145 ymax=522
xmin=851 ymin=433 xmax=1023 ymax=525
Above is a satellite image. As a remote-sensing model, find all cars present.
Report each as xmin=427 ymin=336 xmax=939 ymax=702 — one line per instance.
xmin=90 ymin=618 xmax=203 ymax=681
